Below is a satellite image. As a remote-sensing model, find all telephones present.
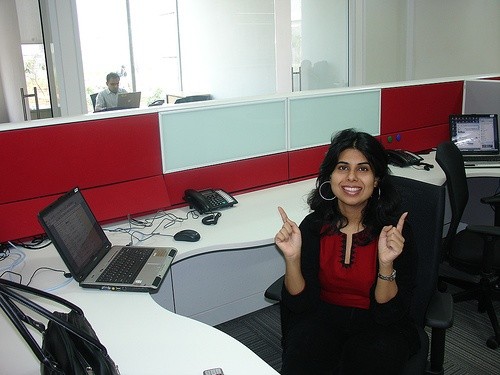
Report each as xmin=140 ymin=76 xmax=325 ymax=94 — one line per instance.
xmin=385 ymin=149 xmax=422 ymax=168
xmin=183 ymin=187 xmax=237 ymax=215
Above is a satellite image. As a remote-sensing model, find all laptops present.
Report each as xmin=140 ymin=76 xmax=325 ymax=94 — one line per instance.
xmin=37 ymin=186 xmax=178 ymax=294
xmin=117 ymin=92 xmax=141 ymax=110
xmin=447 ymin=114 xmax=500 ymax=168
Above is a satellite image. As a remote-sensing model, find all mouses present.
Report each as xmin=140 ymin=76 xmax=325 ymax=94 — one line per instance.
xmin=173 ymin=229 xmax=201 ymax=242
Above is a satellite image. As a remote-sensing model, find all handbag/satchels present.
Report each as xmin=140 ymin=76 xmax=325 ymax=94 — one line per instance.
xmin=0 ymin=278 xmax=119 ymax=375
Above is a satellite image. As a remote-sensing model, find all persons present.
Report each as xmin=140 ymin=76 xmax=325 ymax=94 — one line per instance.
xmin=94 ymin=72 xmax=128 ymax=112
xmin=274 ymin=129 xmax=425 ymax=375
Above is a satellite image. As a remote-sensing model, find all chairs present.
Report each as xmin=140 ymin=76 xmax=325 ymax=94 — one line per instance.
xmin=435 ymin=141 xmax=500 ymax=351
xmin=264 ymin=174 xmax=453 ymax=375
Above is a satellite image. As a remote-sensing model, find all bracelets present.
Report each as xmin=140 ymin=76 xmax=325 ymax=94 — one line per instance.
xmin=378 ymin=269 xmax=397 ymax=281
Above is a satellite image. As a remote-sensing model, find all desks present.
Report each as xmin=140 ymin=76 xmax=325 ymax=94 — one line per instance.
xmin=0 ymin=152 xmax=500 ymax=375
